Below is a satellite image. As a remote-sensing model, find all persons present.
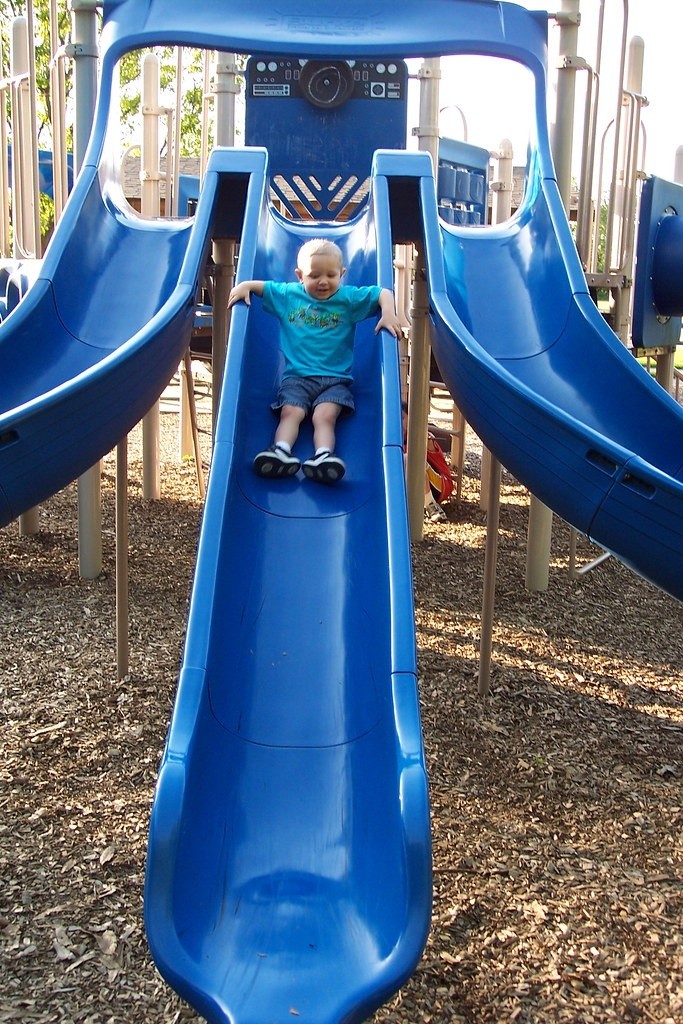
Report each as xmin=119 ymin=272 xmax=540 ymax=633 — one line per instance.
xmin=228 ymin=239 xmax=402 ymax=483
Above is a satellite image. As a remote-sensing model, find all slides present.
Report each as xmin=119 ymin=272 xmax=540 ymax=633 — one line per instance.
xmin=0 ymin=149 xmax=683 ymax=1024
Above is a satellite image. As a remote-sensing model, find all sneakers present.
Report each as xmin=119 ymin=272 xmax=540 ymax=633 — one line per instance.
xmin=254 ymin=446 xmax=300 ymax=478
xmin=303 ymin=452 xmax=346 ymax=484
xmin=426 ymin=502 xmax=447 ymax=522
